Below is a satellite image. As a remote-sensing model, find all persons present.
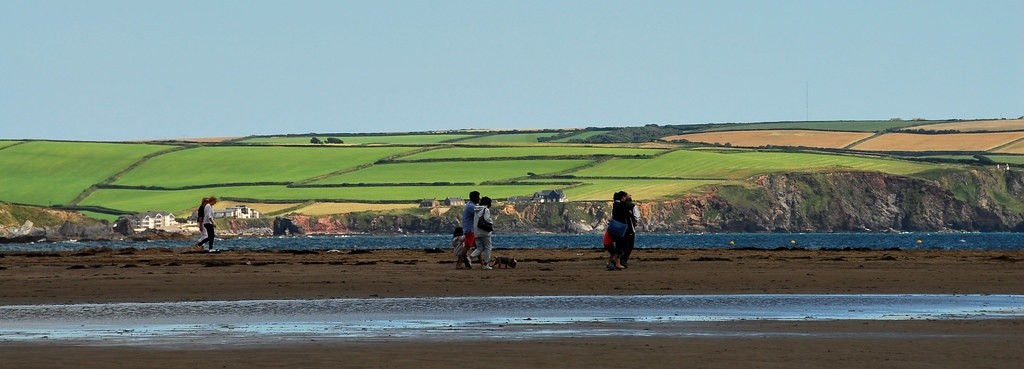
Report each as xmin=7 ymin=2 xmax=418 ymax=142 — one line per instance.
xmin=451 ymin=227 xmax=472 ymax=269
xmin=196 ymin=198 xmax=209 ymax=246
xmin=603 ymin=191 xmax=640 ymax=269
xmin=464 ymin=196 xmax=494 ymax=269
xmin=455 ymin=191 xmax=486 ymax=270
xmin=195 ymin=196 xmax=220 ymax=251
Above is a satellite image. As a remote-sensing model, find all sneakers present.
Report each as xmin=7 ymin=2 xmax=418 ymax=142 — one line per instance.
xmin=464 ymin=256 xmax=472 ymax=269
xmin=481 ymin=264 xmax=493 ymax=270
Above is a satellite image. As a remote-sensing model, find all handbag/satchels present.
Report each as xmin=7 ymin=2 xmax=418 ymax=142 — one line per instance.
xmin=607 ymin=219 xmax=628 ymax=237
xmin=603 ymin=230 xmax=613 ymax=248
xmin=477 ymin=207 xmax=494 ymax=232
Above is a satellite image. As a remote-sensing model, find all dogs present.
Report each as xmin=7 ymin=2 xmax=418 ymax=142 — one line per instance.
xmin=492 ymin=255 xmax=516 ymax=270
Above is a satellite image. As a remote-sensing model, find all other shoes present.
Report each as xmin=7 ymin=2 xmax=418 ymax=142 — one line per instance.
xmin=456 ymin=266 xmax=463 ymax=269
xmin=615 ymin=265 xmax=629 ymax=271
